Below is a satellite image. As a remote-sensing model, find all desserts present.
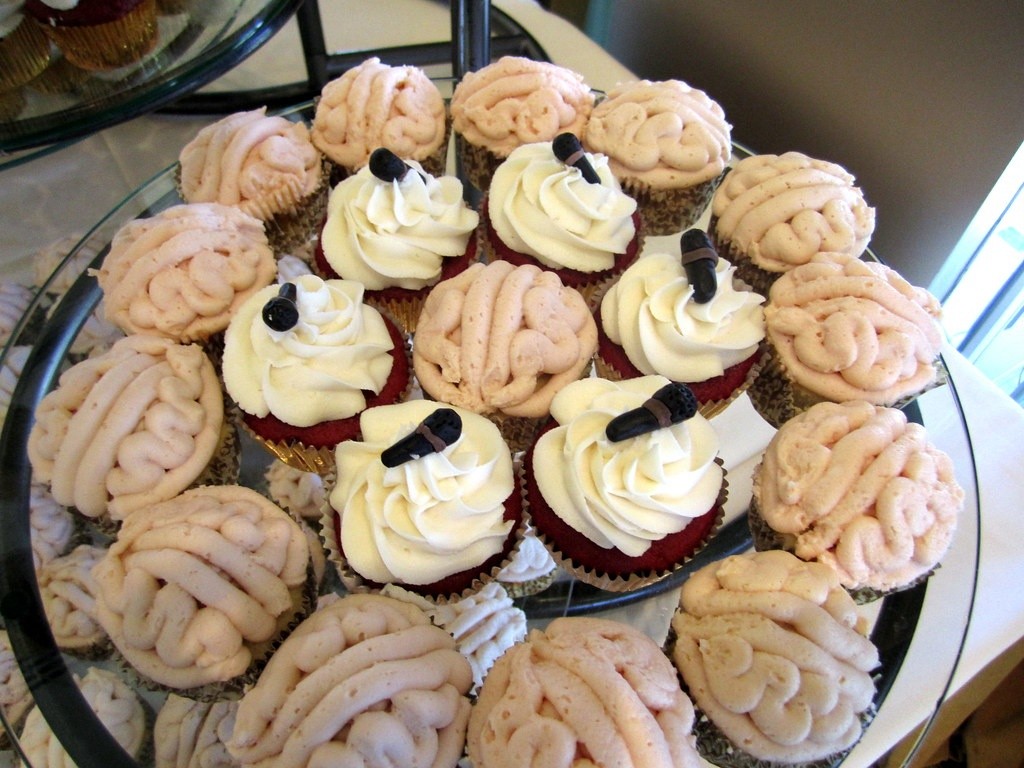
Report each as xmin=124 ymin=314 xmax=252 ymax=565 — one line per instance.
xmin=0 ymin=0 xmax=966 ymax=768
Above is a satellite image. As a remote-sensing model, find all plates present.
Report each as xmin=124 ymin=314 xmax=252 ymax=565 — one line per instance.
xmin=0 ymin=97 xmax=980 ymax=768
